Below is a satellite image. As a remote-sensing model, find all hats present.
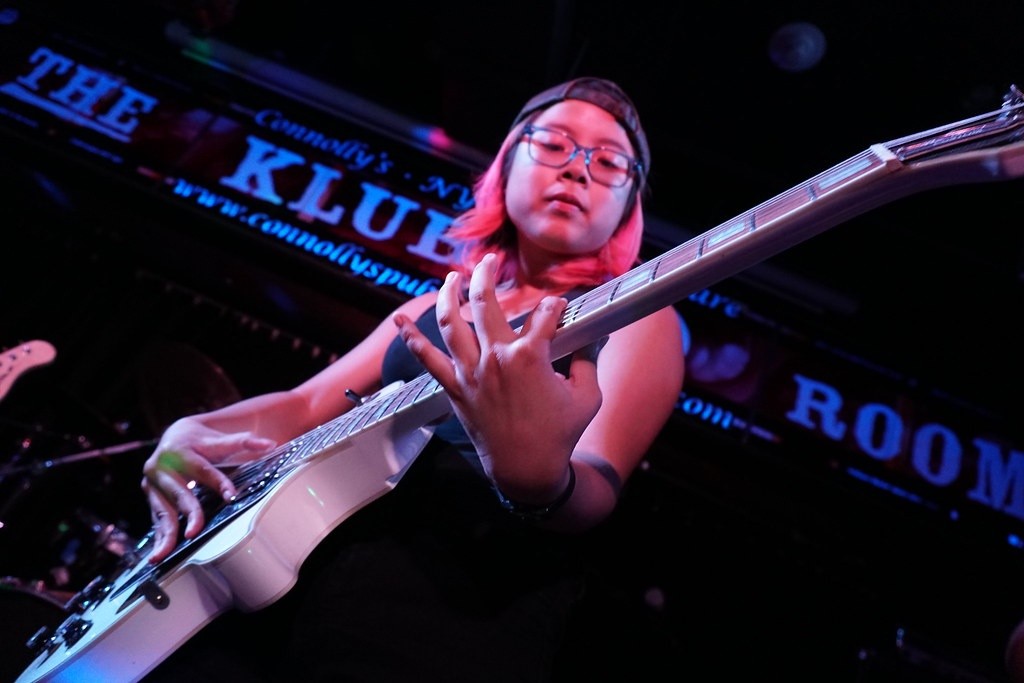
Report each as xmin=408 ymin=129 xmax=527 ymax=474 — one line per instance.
xmin=515 ymin=76 xmax=652 ymax=178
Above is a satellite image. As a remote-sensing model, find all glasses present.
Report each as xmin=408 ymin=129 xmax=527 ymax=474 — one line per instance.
xmin=518 ymin=123 xmax=646 ymax=190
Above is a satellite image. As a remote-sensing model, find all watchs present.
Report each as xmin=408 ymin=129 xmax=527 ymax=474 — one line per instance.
xmin=498 ymin=458 xmax=575 ymax=523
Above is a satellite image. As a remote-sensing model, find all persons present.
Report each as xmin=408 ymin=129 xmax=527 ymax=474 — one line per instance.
xmin=138 ymin=80 xmax=685 ymax=682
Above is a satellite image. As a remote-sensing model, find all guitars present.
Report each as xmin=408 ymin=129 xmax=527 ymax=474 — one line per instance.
xmin=1 ymin=338 xmax=54 ymax=405
xmin=13 ymin=81 xmax=1024 ymax=682
